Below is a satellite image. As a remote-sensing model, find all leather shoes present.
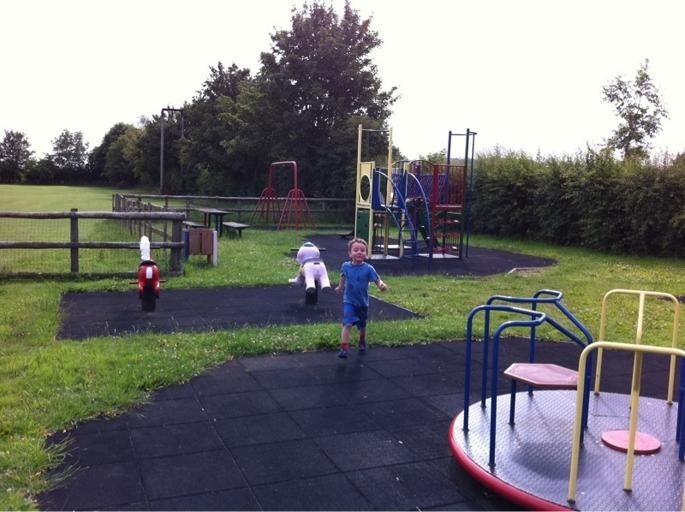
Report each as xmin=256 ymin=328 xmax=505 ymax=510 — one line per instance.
xmin=358 ymin=343 xmax=366 ymax=351
xmin=338 ymin=348 xmax=349 ymax=358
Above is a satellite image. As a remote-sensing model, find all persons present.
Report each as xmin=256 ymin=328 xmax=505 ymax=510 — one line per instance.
xmin=335 ymin=239 xmax=388 ymax=359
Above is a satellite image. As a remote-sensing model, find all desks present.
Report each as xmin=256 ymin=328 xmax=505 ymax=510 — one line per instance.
xmin=191 ymin=208 xmax=233 ymax=237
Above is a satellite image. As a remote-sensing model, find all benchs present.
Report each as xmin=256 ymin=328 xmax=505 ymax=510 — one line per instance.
xmin=183 ymin=220 xmax=206 ymax=229
xmin=222 ymin=221 xmax=251 ymax=238
